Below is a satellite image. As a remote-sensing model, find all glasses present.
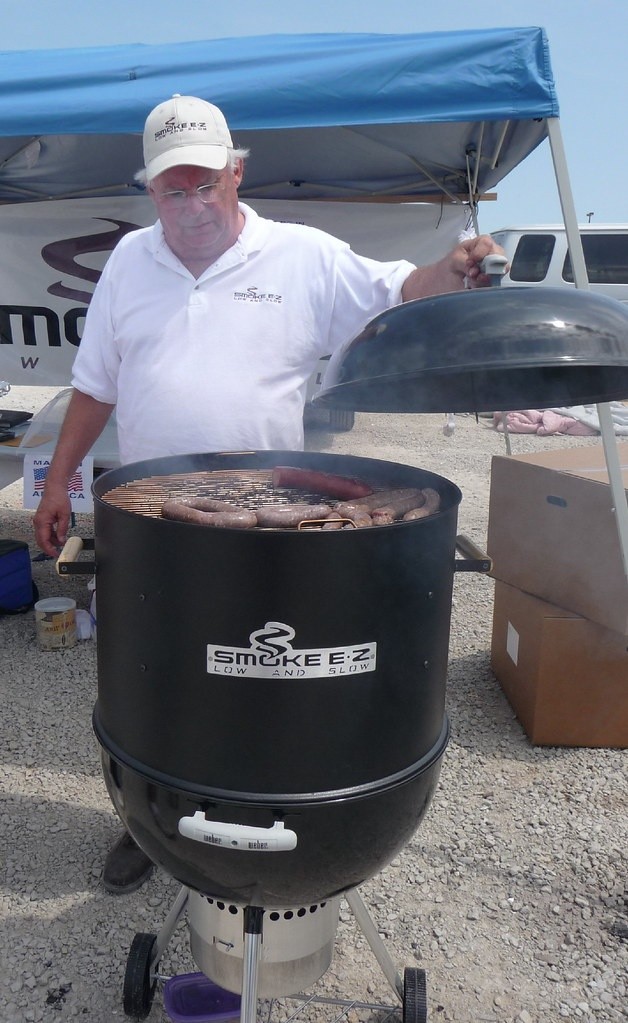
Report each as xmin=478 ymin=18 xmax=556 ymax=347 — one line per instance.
xmin=152 ymin=166 xmax=234 ymax=212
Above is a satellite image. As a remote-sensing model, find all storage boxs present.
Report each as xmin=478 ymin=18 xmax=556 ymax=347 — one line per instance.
xmin=490 ymin=579 xmax=628 ymax=748
xmin=487 ymin=442 xmax=628 ymax=637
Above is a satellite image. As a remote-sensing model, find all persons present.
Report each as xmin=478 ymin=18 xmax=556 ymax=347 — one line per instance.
xmin=33 ymin=91 xmax=512 ymax=893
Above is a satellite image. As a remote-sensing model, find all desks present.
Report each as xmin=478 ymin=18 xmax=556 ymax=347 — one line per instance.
xmin=0 ymin=420 xmax=121 ymax=490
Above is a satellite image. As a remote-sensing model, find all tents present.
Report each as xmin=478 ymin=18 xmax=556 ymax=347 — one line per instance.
xmin=0 ymin=25 xmax=628 ymax=578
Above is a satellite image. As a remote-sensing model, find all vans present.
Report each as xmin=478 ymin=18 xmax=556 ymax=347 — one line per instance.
xmin=488 ymin=224 xmax=627 ymax=306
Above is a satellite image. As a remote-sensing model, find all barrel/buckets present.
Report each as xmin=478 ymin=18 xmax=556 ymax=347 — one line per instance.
xmin=35 ymin=597 xmax=76 ymax=651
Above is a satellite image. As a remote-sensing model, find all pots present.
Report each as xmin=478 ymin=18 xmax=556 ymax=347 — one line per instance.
xmin=56 ymin=450 xmax=490 ymax=903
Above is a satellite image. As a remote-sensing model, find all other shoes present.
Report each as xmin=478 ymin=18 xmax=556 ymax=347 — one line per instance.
xmin=100 ymin=827 xmax=154 ymax=894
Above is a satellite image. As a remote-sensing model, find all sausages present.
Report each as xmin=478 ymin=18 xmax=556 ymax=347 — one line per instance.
xmin=160 ymin=487 xmax=441 ymax=530
xmin=271 ymin=468 xmax=374 ymax=500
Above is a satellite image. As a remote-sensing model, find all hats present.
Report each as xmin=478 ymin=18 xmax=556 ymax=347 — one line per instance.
xmin=143 ymin=92 xmax=234 ymax=183
